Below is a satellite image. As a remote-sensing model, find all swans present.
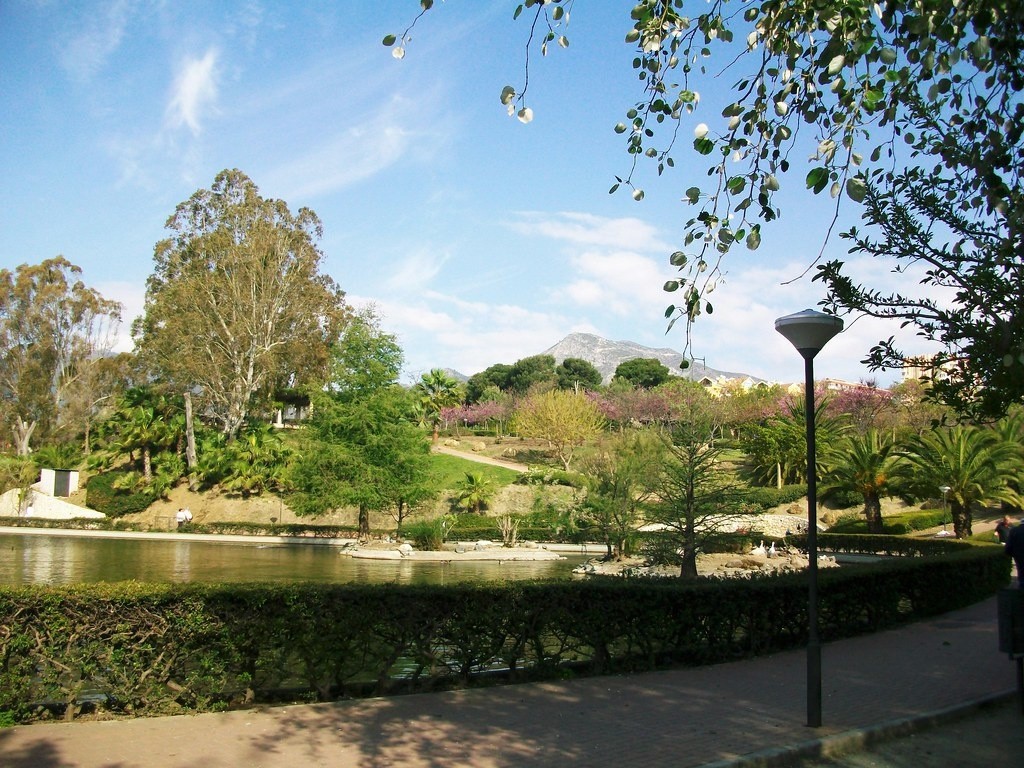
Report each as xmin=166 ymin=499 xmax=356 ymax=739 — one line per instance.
xmin=749 ymin=539 xmax=775 ymax=558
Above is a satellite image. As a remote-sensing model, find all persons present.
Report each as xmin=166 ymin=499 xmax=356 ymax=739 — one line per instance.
xmin=177 ymin=509 xmax=185 ymax=528
xmin=184 ymin=506 xmax=193 ymax=524
xmin=442 ymin=513 xmax=449 ymax=539
xmin=1004 ymin=518 xmax=1024 ymax=590
xmin=993 ymin=515 xmax=1016 ymax=546
